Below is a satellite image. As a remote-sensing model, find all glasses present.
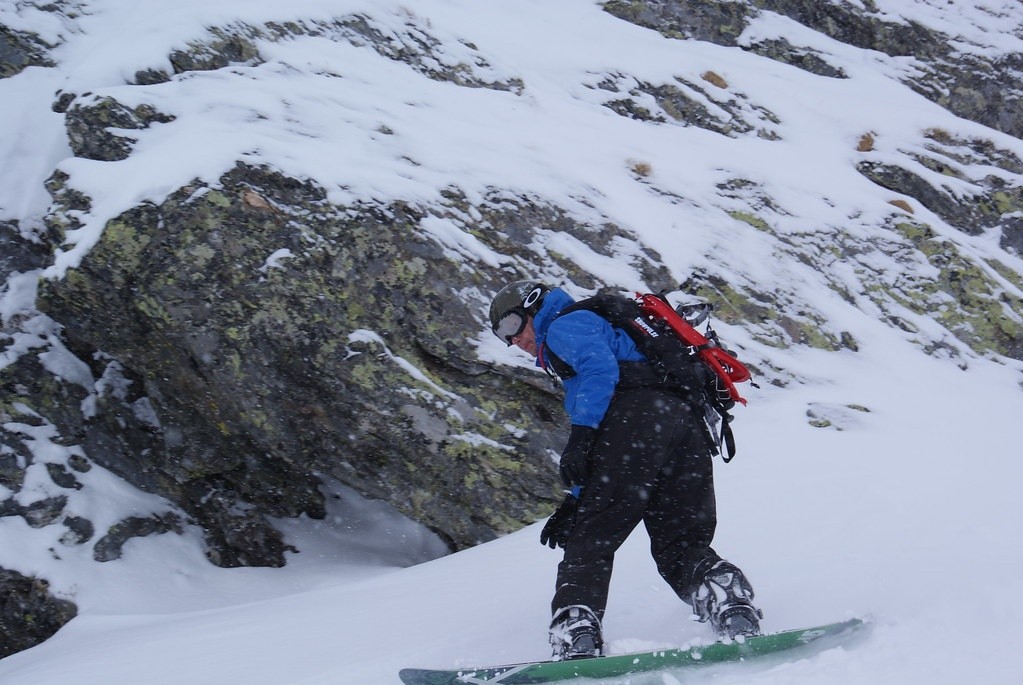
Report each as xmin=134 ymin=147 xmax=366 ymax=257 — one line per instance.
xmin=491 ymin=306 xmax=527 ymax=347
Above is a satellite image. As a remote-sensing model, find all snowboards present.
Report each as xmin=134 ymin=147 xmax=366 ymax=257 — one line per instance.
xmin=399 ymin=618 xmax=867 ymax=684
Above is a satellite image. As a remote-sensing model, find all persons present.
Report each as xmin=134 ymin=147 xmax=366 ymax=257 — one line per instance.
xmin=490 ymin=279 xmax=764 ymax=661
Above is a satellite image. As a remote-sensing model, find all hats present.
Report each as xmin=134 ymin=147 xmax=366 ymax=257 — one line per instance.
xmin=490 ymin=280 xmax=539 ymax=325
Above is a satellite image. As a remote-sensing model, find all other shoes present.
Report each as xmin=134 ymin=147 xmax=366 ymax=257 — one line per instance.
xmin=549 ymin=605 xmax=603 ymax=658
xmin=705 ymin=572 xmax=763 ymax=640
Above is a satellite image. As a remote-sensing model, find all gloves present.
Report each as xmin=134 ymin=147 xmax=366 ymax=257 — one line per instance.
xmin=559 ymin=424 xmax=596 ymax=488
xmin=540 ymin=493 xmax=580 ymax=549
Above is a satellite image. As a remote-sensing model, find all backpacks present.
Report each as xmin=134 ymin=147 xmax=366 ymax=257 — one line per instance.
xmin=539 ymin=285 xmax=748 ymax=415
